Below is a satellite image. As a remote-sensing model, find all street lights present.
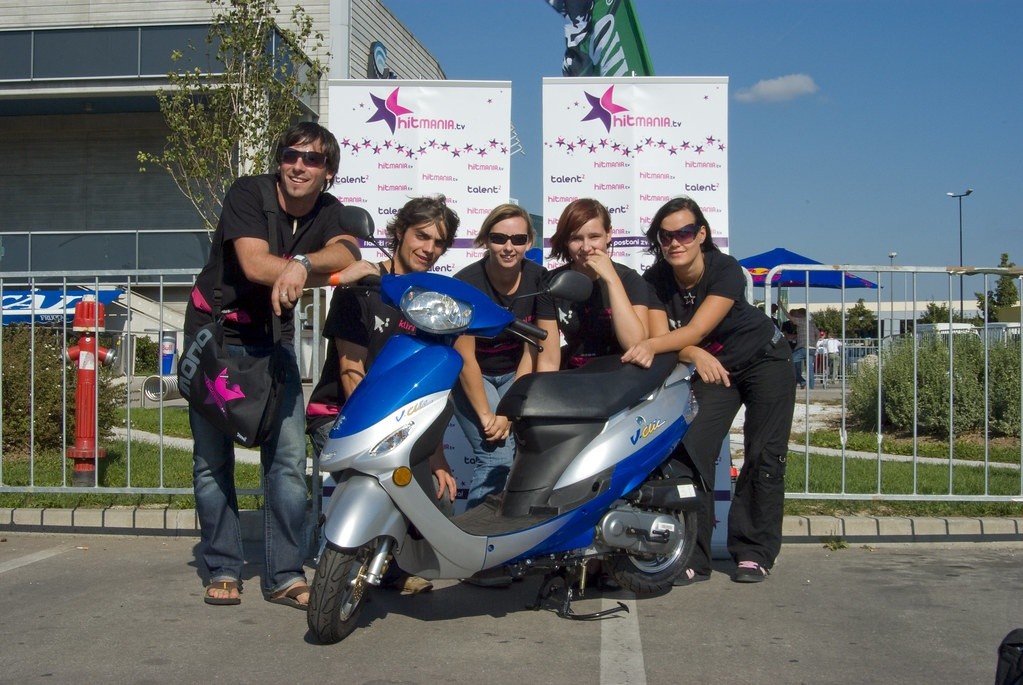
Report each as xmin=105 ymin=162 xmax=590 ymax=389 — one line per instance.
xmin=887 ymin=251 xmax=897 ymax=361
xmin=945 ymin=187 xmax=973 ymax=323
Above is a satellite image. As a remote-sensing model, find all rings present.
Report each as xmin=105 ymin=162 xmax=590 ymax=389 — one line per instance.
xmin=280 ymin=291 xmax=287 ymax=296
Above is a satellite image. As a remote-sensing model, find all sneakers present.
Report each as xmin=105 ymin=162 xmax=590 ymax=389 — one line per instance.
xmin=672 ymin=568 xmax=710 ymax=586
xmin=735 ymin=560 xmax=767 ymax=582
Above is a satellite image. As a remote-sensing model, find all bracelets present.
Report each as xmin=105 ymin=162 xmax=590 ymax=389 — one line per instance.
xmin=331 ymin=273 xmax=338 ymax=286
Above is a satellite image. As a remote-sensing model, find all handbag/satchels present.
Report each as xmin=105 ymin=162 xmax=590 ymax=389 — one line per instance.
xmin=177 ymin=323 xmax=289 ymax=448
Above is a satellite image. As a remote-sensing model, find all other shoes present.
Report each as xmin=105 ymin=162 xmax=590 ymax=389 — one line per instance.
xmin=392 ymin=573 xmax=432 ymax=593
xmin=462 ymin=573 xmax=512 ymax=586
xmin=588 ymin=569 xmax=622 ymax=593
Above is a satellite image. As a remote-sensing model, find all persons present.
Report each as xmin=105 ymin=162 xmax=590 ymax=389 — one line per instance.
xmin=307 ymin=197 xmax=460 ymax=598
xmin=453 ymin=203 xmax=549 ymax=587
xmin=771 ymin=304 xmax=781 ymax=330
xmin=621 ymin=199 xmax=794 ymax=590
xmin=535 ymin=197 xmax=650 ymax=592
xmin=183 ymin=120 xmax=381 ymax=611
xmin=814 ymin=332 xmax=841 ymax=384
xmin=777 ymin=301 xmax=820 ymax=388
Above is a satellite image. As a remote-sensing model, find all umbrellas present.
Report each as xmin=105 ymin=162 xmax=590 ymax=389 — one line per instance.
xmin=737 ymin=247 xmax=884 ymax=326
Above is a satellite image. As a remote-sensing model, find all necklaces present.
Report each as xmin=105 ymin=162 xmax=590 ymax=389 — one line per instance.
xmin=293 ymin=219 xmax=297 ymax=235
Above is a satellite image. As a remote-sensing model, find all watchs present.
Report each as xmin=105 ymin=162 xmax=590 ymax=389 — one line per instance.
xmin=293 ymin=255 xmax=311 ymax=272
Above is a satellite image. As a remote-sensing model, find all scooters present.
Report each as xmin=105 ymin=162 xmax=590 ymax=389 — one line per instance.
xmin=306 ymin=205 xmax=700 ymax=645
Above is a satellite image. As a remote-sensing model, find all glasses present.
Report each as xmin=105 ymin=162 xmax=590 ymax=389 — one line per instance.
xmin=280 ymin=147 xmax=327 ymax=167
xmin=489 ymin=233 xmax=527 ymax=245
xmin=656 ymin=224 xmax=700 ymax=246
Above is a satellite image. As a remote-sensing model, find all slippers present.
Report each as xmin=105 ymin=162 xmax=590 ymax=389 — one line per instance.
xmin=270 ymin=585 xmax=312 ymax=610
xmin=203 ymin=581 xmax=241 ymax=605
